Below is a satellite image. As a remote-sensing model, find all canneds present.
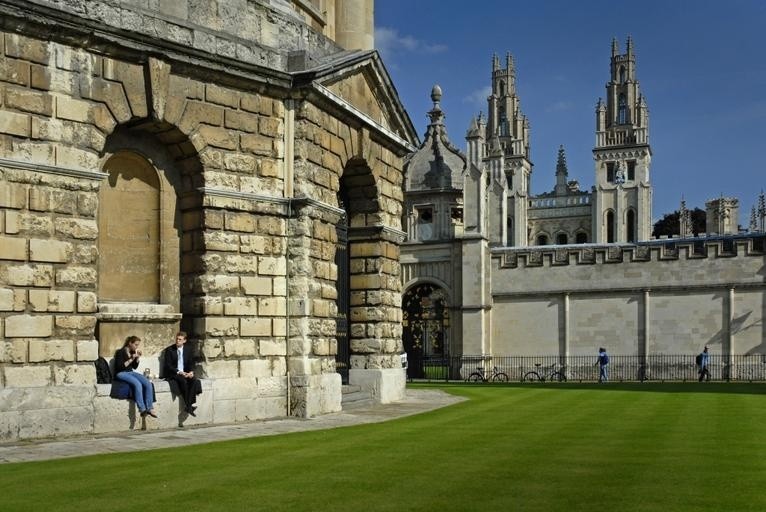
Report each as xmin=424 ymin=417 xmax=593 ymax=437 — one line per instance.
xmin=145 ymin=368 xmax=150 ymax=375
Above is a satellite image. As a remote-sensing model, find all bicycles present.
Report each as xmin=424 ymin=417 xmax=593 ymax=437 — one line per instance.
xmin=522 ymin=361 xmax=566 ymax=382
xmin=466 ymin=365 xmax=509 ymax=382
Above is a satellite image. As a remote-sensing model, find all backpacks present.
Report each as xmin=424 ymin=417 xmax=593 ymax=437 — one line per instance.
xmin=600 ymin=352 xmax=609 ymax=364
xmin=94 ymin=357 xmax=112 ymax=384
xmin=696 ymin=354 xmax=702 ymax=365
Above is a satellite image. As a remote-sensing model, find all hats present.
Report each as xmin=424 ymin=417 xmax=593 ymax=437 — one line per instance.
xmin=706 ymin=345 xmax=710 ymax=349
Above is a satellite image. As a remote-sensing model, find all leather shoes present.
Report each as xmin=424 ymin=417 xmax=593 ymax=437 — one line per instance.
xmin=186 ymin=407 xmax=197 ymax=413
xmin=185 ymin=407 xmax=196 ymax=417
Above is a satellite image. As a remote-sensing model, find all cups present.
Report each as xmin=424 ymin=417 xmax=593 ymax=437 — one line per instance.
xmin=144 ymin=368 xmax=150 ymax=379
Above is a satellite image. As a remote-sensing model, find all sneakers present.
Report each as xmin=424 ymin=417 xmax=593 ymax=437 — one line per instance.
xmin=141 ymin=409 xmax=147 ymax=417
xmin=148 ymin=409 xmax=158 ymax=418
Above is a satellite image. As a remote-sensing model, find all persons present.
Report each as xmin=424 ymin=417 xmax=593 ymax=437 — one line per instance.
xmin=114 ymin=335 xmax=158 ymax=419
xmin=163 ymin=331 xmax=202 ymax=417
xmin=594 ymin=347 xmax=609 ymax=382
xmin=696 ymin=345 xmax=711 ymax=382
